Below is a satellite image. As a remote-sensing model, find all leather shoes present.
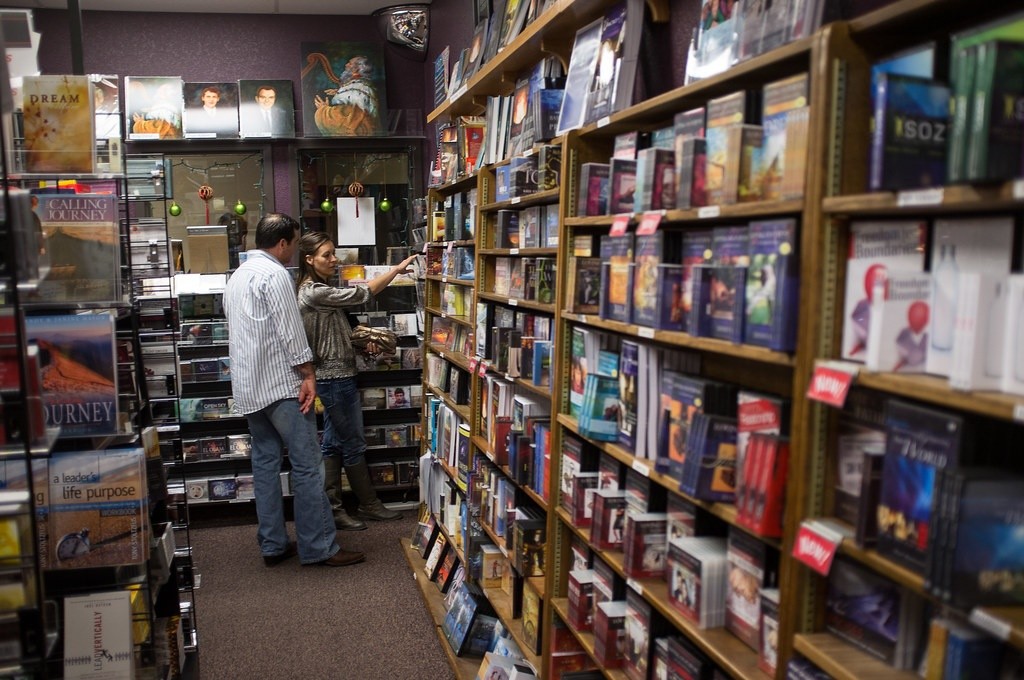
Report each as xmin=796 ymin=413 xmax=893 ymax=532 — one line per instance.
xmin=261 ymin=541 xmax=297 ymax=567
xmin=324 ymin=549 xmax=365 ymax=566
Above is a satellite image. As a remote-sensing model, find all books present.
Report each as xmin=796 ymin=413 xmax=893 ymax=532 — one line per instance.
xmin=2 ymin=0 xmax=1024 ymax=680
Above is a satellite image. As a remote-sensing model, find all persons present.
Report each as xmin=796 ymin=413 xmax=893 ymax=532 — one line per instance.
xmin=129 ymin=82 xmax=181 ymax=139
xmin=296 ymin=232 xmax=419 ymax=530
xmin=198 ymin=87 xmax=221 ymax=113
xmin=222 ymin=212 xmax=364 ymax=567
xmin=389 ymin=388 xmax=411 ymax=408
xmin=246 ymin=85 xmax=291 ymax=135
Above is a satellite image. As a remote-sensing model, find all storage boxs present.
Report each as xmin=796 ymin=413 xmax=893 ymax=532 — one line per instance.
xmin=479 ymin=544 xmax=503 ymax=589
xmin=449 ymin=593 xmax=508 ymax=659
xmin=521 ymin=581 xmax=544 ymax=656
xmin=441 ymin=581 xmax=484 ymax=639
xmin=180 ymin=235 xmax=228 ymax=273
xmin=23 ymin=75 xmax=97 ymax=173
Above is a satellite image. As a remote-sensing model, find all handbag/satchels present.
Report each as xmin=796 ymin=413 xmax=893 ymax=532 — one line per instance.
xmin=349 ymin=326 xmax=397 ymax=354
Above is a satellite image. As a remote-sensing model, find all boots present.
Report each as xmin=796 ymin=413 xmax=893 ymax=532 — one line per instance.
xmin=344 ymin=459 xmax=403 ymax=521
xmin=323 ymin=456 xmax=368 ymax=531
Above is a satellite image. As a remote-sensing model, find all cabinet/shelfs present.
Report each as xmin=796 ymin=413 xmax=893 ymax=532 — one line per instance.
xmin=0 ymin=0 xmax=293 ymax=680
xmin=301 ymin=0 xmax=1024 ymax=680
